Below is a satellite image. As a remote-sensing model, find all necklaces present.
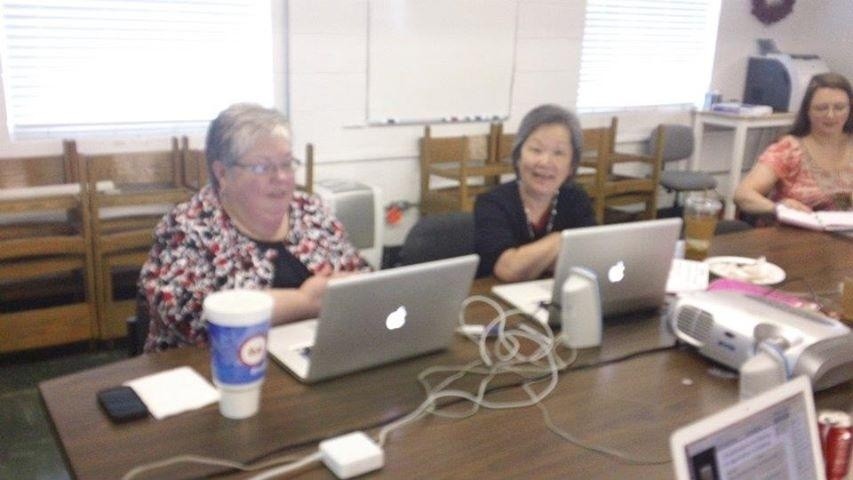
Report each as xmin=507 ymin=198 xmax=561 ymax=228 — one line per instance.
xmin=517 ymin=188 xmax=557 ymax=241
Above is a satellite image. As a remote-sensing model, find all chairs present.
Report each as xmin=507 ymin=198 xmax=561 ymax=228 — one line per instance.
xmin=649 ymin=122 xmax=722 ymax=212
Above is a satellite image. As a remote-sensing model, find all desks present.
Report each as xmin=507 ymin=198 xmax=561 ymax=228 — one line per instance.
xmin=37 ymin=224 xmax=853 ymax=480
xmin=691 ymin=108 xmax=798 ymax=221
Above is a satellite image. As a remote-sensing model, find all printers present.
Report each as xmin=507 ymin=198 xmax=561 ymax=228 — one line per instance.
xmin=741 ymin=39 xmax=831 ymax=113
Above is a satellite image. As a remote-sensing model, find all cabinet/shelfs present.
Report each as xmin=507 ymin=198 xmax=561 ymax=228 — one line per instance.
xmin=418 ymin=117 xmax=666 ymax=225
xmin=0 ymin=140 xmax=314 ymax=354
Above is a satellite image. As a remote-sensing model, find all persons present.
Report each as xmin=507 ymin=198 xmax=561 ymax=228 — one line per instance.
xmin=733 ymin=73 xmax=852 ymax=227
xmin=134 ymin=102 xmax=373 ymax=354
xmin=474 ymin=105 xmax=600 ymax=283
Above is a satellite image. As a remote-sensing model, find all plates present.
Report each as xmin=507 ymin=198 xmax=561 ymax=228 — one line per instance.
xmin=664 ymin=257 xmax=708 ymax=292
xmin=703 ymin=256 xmax=787 ymax=285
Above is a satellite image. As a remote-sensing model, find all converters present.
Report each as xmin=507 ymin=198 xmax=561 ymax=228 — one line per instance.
xmin=316 ymin=431 xmax=387 ymax=478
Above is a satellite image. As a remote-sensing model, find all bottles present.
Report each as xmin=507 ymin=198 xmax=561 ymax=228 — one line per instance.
xmin=819 ymin=408 xmax=853 ymax=480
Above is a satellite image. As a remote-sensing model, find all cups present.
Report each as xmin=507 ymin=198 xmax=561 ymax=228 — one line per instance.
xmin=205 ymin=290 xmax=273 ymax=419
xmin=683 ymin=198 xmax=722 ymax=259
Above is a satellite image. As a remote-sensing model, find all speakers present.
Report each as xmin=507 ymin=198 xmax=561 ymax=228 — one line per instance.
xmin=560 ymin=266 xmax=601 ymax=346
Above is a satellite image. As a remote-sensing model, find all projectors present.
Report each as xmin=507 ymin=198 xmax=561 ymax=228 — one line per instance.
xmin=667 ymin=289 xmax=852 ymax=393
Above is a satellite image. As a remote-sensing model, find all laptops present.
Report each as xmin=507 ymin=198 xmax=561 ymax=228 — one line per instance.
xmin=490 ymin=216 xmax=683 ymax=328
xmin=669 ymin=375 xmax=827 ymax=480
xmin=267 ymin=253 xmax=480 ymax=382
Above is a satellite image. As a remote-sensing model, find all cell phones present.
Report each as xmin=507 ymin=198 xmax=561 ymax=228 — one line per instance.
xmin=98 ymin=386 xmax=148 ymax=424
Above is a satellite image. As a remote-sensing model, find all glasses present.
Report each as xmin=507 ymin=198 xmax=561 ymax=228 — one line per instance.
xmin=229 ymin=157 xmax=303 ymax=176
